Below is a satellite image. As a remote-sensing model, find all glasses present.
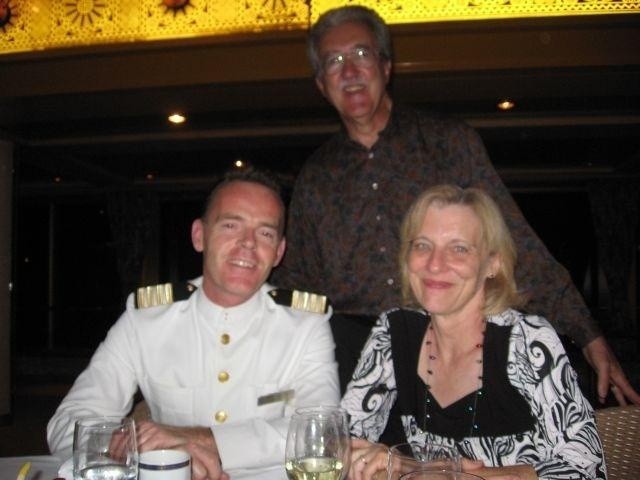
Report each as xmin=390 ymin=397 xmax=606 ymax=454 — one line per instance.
xmin=318 ymin=46 xmax=383 ymax=71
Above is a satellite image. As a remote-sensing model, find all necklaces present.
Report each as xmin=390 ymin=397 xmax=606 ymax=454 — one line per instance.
xmin=421 ymin=319 xmax=487 ymax=464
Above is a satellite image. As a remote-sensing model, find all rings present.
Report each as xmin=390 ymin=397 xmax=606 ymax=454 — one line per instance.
xmin=610 ymin=385 xmax=618 ymax=393
xmin=360 ymin=456 xmax=367 ymax=463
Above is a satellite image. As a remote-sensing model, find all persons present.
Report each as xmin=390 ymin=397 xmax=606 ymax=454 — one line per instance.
xmin=46 ymin=166 xmax=342 ymax=479
xmin=325 ymin=183 xmax=608 ymax=479
xmin=263 ymin=4 xmax=639 ymax=410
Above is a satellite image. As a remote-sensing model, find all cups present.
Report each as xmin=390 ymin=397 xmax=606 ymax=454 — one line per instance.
xmin=72 ymin=414 xmax=192 ymax=479
xmin=284 ymin=406 xmax=463 ymax=479
